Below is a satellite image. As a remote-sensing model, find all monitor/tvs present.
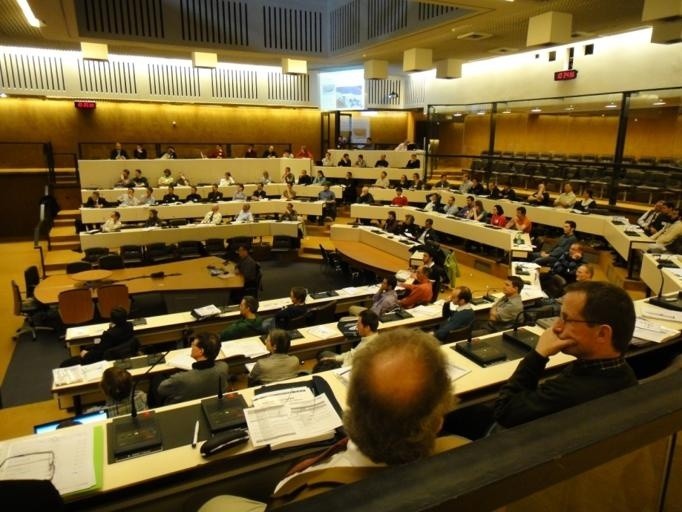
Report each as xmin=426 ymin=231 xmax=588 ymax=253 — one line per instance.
xmin=32 ymin=412 xmax=111 ymax=434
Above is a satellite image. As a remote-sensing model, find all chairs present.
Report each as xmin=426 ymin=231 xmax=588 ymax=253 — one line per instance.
xmin=462 ymin=150 xmax=682 ymax=211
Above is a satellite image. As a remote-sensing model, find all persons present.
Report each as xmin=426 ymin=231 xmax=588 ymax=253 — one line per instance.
xmin=81 ymin=142 xmax=682 ymax=512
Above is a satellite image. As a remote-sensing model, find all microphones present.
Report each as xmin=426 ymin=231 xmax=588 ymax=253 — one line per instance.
xmin=455 ymin=318 xmax=507 ymax=366
xmin=503 ymin=307 xmax=554 ymax=354
xmin=647 ymin=264 xmax=682 ymax=311
xmin=201 ymin=374 xmax=250 ymax=434
xmin=112 ymin=328 xmax=195 ymax=463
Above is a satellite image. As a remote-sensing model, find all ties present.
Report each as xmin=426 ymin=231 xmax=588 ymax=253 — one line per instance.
xmin=278 ymin=434 xmax=349 ymax=480
xmin=209 ymin=212 xmax=214 ymax=222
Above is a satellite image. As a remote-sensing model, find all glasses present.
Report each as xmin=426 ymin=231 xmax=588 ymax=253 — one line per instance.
xmin=559 ymin=311 xmax=613 ymax=325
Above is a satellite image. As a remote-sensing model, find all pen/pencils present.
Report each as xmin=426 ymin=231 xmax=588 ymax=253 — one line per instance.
xmin=193 ymin=419 xmax=200 ymax=447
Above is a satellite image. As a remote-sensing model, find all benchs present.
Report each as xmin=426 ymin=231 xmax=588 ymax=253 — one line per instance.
xmin=77 ymin=158 xmax=344 ymax=251
xmin=312 ymin=149 xmax=433 ymax=179
